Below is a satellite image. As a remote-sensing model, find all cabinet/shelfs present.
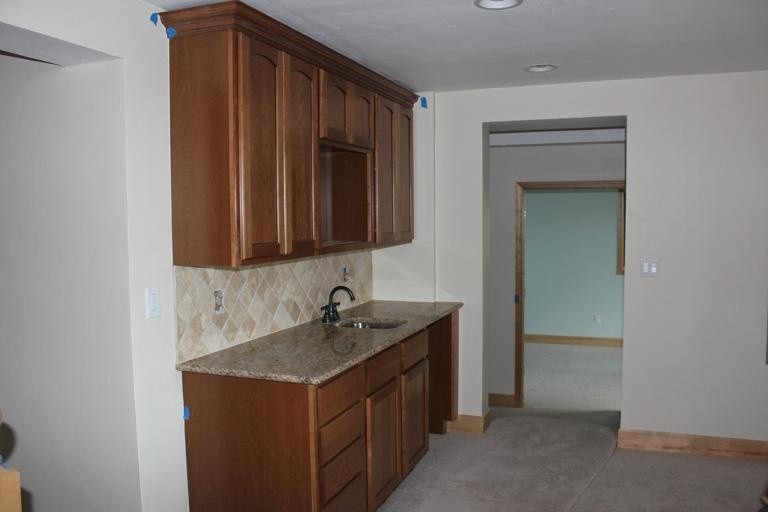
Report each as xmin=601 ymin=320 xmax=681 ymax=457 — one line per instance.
xmin=156 ymin=1 xmax=321 ymax=274
xmin=320 ymin=43 xmax=379 ymax=261
xmin=402 ymin=326 xmax=432 ymax=483
xmin=431 ymin=307 xmax=464 ymax=435
xmin=363 ymin=346 xmax=407 ymax=512
xmin=371 ymin=67 xmax=420 ymax=252
xmin=182 ymin=359 xmax=369 ymax=512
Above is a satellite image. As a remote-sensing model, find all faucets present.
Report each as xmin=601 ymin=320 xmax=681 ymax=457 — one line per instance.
xmin=329 ymin=286 xmax=355 ymax=321
xmin=329 ymin=324 xmax=357 ymax=358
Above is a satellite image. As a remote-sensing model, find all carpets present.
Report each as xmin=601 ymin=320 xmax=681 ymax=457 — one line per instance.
xmin=566 ymin=442 xmax=766 ymax=512
xmin=375 ymin=408 xmax=616 ymax=512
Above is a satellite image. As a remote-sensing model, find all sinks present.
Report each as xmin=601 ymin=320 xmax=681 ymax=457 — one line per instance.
xmin=334 ymin=317 xmax=409 ymax=329
